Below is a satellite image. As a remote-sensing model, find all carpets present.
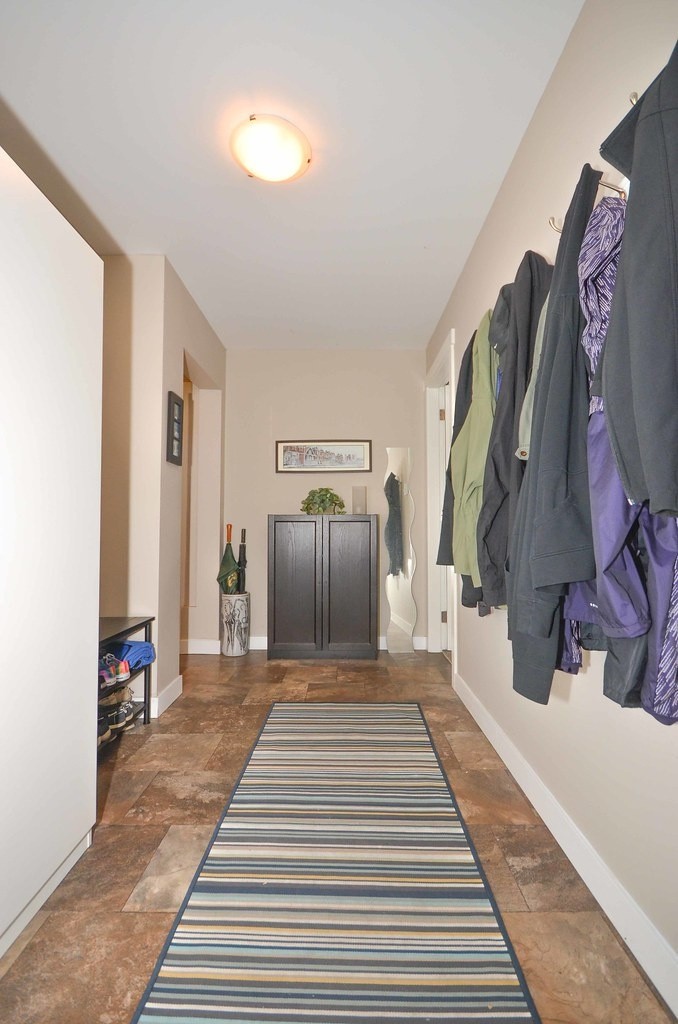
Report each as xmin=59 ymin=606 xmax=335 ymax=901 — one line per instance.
xmin=130 ymin=701 xmax=541 ymax=1024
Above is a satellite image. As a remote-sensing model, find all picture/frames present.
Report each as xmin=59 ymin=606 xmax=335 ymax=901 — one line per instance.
xmin=275 ymin=439 xmax=373 ymax=473
xmin=165 ymin=390 xmax=184 ymax=466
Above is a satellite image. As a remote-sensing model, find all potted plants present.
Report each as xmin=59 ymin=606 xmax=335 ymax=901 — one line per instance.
xmin=300 ymin=488 xmax=346 ymax=515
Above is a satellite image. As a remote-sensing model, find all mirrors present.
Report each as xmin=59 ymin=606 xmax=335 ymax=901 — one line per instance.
xmin=383 ymin=447 xmax=417 ymax=655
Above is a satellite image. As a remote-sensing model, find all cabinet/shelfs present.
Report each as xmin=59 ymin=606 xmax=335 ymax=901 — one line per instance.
xmin=99 ymin=616 xmax=156 ymax=751
xmin=265 ymin=514 xmax=380 ymax=660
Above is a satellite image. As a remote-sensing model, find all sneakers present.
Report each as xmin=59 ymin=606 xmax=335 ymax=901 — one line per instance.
xmin=98 ymin=674 xmax=135 ymax=747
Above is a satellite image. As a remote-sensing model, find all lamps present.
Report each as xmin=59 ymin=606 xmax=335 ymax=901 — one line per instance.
xmin=233 ymin=113 xmax=312 ymax=182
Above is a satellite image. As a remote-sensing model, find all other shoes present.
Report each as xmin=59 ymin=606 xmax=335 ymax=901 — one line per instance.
xmin=98 ymin=653 xmax=131 ymax=687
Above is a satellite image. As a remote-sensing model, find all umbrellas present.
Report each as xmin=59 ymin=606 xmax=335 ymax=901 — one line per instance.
xmin=217 ymin=523 xmax=241 ymax=595
xmin=236 ymin=528 xmax=248 ymax=594
